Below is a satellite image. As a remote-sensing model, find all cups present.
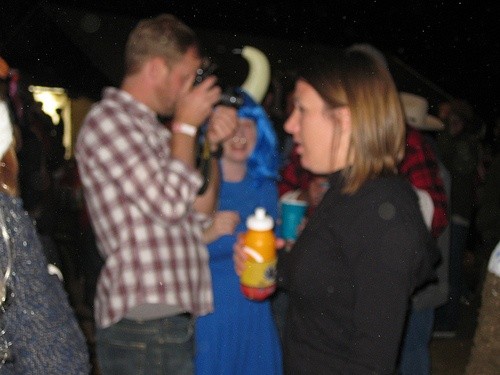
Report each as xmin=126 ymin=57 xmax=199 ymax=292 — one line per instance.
xmin=281 ymin=200 xmax=309 ymax=242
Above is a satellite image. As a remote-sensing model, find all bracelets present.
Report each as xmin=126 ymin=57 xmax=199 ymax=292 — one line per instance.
xmin=171 ymin=120 xmax=198 ymax=136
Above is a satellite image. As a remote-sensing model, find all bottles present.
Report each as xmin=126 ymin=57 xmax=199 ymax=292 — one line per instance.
xmin=241 ymin=208 xmax=278 ymax=299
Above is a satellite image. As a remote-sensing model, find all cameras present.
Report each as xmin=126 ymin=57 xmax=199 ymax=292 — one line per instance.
xmin=195 ymin=53 xmax=250 ymax=108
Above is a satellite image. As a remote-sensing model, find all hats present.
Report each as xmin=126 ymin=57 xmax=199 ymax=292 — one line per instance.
xmin=399 ymin=91 xmax=445 ymax=130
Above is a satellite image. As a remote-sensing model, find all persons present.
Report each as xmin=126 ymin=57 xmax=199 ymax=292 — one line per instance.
xmin=75 ymin=15 xmax=239 ymax=375
xmin=0 ymin=58 xmax=103 ymax=375
xmin=234 ymin=45 xmax=437 ymax=374
xmin=192 ymin=91 xmax=309 ymax=374
xmin=264 ymin=77 xmax=500 ymax=374
